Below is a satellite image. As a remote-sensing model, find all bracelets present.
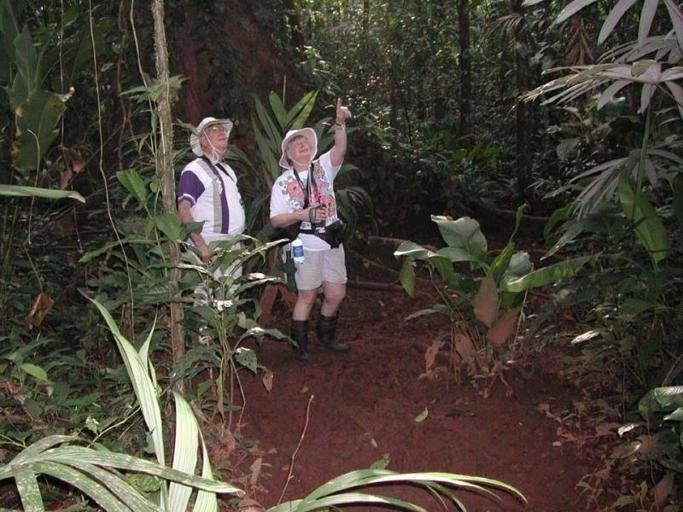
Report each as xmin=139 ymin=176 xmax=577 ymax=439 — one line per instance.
xmin=333 ymin=118 xmax=348 ymax=129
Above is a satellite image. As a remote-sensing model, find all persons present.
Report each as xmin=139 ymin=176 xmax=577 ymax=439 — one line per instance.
xmin=176 ymin=116 xmax=246 ymax=380
xmin=270 ymin=96 xmax=355 ymax=364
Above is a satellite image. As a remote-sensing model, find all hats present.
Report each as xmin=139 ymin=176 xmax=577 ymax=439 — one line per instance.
xmin=277 ymin=127 xmax=319 ymax=170
xmin=189 ymin=116 xmax=233 ymax=158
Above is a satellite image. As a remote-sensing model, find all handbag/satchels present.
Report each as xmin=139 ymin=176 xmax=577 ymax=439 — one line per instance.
xmin=269 ymin=218 xmax=304 ymax=246
xmin=298 ymin=219 xmax=352 ymax=250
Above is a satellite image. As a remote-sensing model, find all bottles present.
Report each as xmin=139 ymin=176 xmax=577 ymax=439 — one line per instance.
xmin=290 ymin=236 xmax=304 ymax=264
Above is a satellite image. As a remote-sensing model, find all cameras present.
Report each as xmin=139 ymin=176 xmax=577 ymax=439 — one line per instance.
xmin=313 ymin=203 xmax=327 ymax=227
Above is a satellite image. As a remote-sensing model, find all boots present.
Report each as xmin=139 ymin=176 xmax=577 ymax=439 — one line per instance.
xmin=314 ymin=309 xmax=351 ymax=352
xmin=290 ymin=318 xmax=312 ymax=362
